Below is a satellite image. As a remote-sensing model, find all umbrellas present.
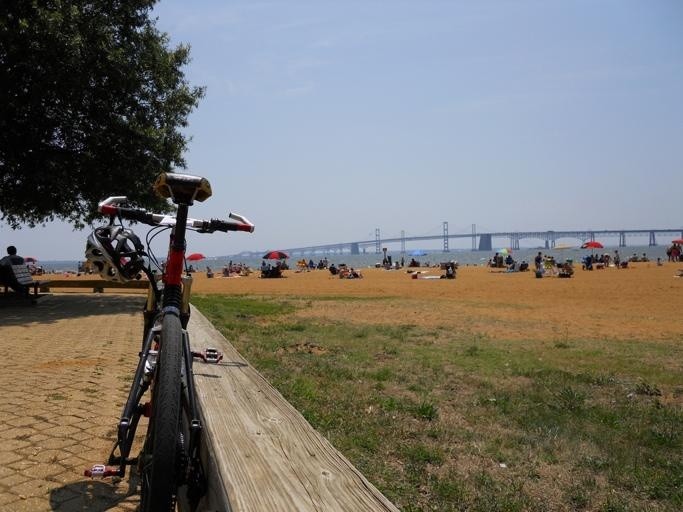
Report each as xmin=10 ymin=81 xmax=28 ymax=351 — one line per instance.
xmin=23 ymin=257 xmax=37 ymax=262
xmin=496 ymin=247 xmax=513 ymax=257
xmin=671 ymin=238 xmax=683 ymax=247
xmin=262 ymin=251 xmax=290 ymax=266
xmin=186 ymin=253 xmax=206 ymax=270
xmin=579 ymin=241 xmax=604 ymax=256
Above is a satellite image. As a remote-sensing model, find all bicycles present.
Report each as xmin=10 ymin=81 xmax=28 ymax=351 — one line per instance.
xmin=84 ymin=171 xmax=256 ymax=512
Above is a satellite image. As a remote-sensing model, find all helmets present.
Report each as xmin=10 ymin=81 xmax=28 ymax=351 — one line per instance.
xmin=85 ymin=224 xmax=144 ymax=284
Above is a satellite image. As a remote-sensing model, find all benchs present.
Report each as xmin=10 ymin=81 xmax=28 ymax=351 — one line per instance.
xmin=7 ymin=264 xmax=48 ymax=298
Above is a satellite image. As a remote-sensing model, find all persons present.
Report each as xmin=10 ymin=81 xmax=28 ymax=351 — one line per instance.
xmin=222 ymin=259 xmax=246 ymax=277
xmin=445 ymin=262 xmax=458 ymax=279
xmin=300 ymin=257 xmax=359 ymax=280
xmin=77 ymin=259 xmax=91 ymax=276
xmin=535 ymin=252 xmax=575 ymax=278
xmin=7 ymin=246 xmax=24 ymax=300
xmin=27 ymin=265 xmax=45 ymax=276
xmin=161 ymin=260 xmax=165 ymax=266
xmin=261 ymin=260 xmax=289 ymax=274
xmin=383 ymin=256 xmax=417 ymax=270
xmin=658 ymin=242 xmax=683 ymax=266
xmin=488 ymin=252 xmax=529 ymax=272
xmin=189 ymin=264 xmax=194 ymax=272
xmin=206 ymin=266 xmax=212 ymax=272
xmin=581 ymin=250 xmax=649 ymax=272
